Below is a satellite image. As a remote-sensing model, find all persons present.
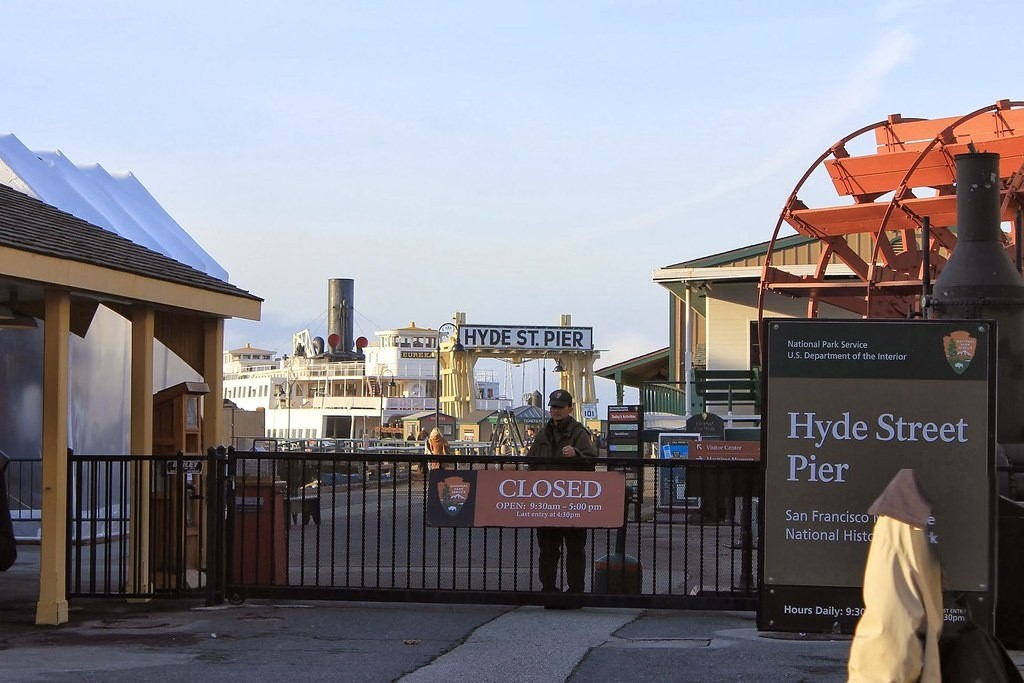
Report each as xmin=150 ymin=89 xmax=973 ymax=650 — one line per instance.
xmin=428 ymin=427 xmax=450 ymax=470
xmin=527 ymin=428 xmax=538 ymax=443
xmin=586 ymin=426 xmax=605 ymax=458
xmin=525 ymin=389 xmax=598 ymax=611
xmin=406 ymin=432 xmax=416 ymax=447
xmin=417 ymin=427 xmax=429 ymax=446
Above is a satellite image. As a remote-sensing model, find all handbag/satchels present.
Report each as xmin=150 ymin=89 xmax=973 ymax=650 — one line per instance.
xmin=442 ymin=437 xmax=451 ymax=455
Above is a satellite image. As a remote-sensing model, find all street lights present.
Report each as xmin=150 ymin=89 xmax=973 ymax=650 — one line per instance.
xmin=279 ymin=381 xmax=291 ymax=439
xmin=542 ymin=349 xmax=567 ymax=429
xmin=435 ymin=322 xmax=466 ymax=427
xmin=379 ymin=369 xmax=397 ymax=440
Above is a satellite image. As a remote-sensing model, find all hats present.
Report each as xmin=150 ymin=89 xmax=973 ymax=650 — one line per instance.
xmin=548 ymin=388 xmax=572 ymax=407
